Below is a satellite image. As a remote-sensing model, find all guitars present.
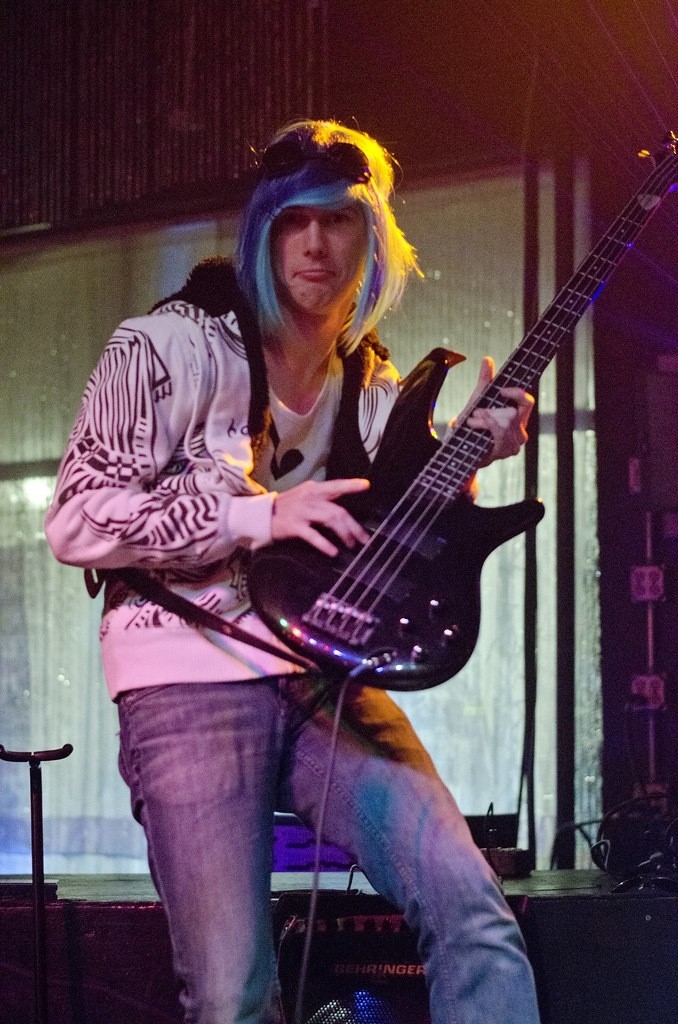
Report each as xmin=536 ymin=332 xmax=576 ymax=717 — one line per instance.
xmin=244 ymin=125 xmax=678 ymax=692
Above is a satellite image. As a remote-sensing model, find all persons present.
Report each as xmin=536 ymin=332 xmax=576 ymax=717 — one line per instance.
xmin=43 ymin=118 xmax=541 ymax=1024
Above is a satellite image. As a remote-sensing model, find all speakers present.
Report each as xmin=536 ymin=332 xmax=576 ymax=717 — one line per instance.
xmin=269 ymin=872 xmax=678 ymax=1024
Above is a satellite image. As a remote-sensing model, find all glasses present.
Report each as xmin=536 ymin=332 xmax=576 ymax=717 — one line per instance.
xmin=257 ymin=140 xmax=367 ymax=180
